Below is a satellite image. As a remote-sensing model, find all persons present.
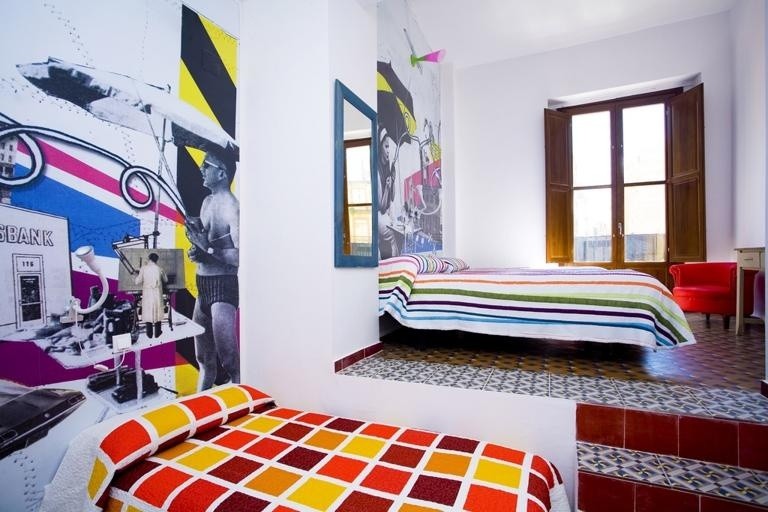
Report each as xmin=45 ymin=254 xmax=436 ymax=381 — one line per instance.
xmin=421 ymin=117 xmax=437 ymax=170
xmin=135 ymin=252 xmax=170 ymax=337
xmin=183 ymin=147 xmax=239 ymax=394
xmin=373 ymin=126 xmax=398 ymax=258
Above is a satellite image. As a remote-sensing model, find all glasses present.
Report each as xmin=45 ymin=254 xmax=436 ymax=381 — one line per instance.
xmin=410 ymin=48 xmax=445 ymax=66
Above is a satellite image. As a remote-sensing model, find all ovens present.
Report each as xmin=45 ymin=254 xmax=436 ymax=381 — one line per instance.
xmin=38 ymin=373 xmax=576 ymax=512
xmin=378 ymin=254 xmax=697 ymax=350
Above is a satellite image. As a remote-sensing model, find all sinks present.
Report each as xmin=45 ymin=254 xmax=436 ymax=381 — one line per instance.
xmin=668 ymin=262 xmax=758 ymax=330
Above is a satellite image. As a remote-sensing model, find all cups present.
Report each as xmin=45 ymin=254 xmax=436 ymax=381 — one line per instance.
xmin=201 ymin=158 xmax=226 ymax=172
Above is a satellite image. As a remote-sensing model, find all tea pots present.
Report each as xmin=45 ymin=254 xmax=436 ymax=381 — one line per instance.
xmin=334 ymin=78 xmax=378 ymax=267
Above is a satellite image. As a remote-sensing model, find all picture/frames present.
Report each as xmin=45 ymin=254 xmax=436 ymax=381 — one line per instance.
xmin=733 ymin=247 xmax=764 ymax=336
